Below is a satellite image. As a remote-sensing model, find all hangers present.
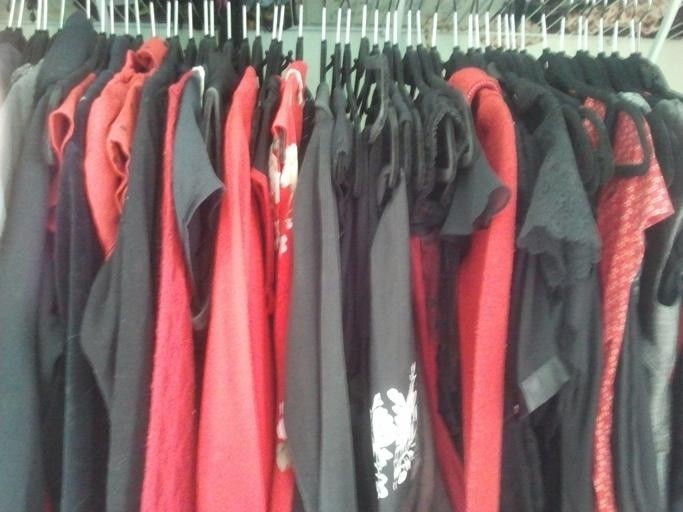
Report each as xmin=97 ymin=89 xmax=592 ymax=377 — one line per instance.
xmin=3 ymin=0 xmax=304 ymax=112
xmin=319 ymin=2 xmax=682 ymax=198
